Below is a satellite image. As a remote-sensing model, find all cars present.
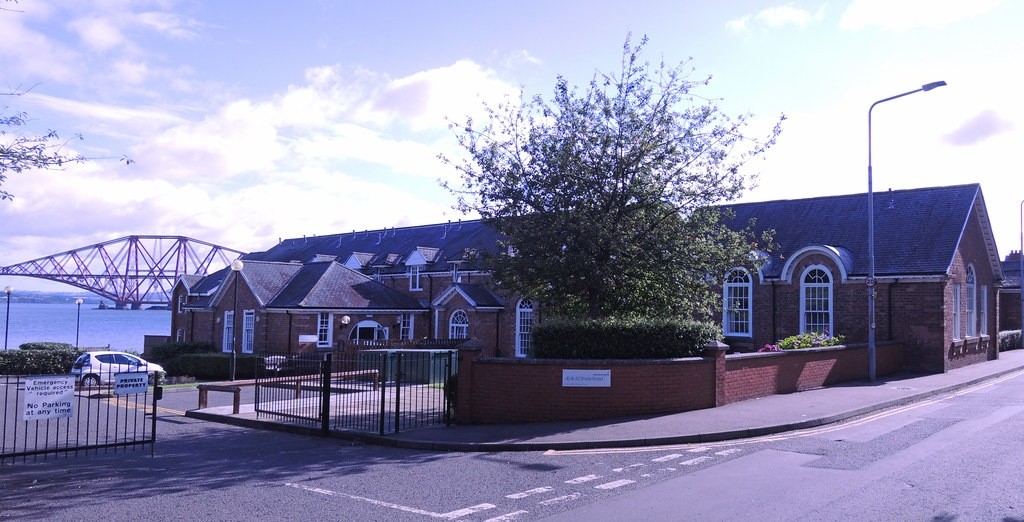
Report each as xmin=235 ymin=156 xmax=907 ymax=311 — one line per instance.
xmin=71 ymin=351 xmax=168 ymax=386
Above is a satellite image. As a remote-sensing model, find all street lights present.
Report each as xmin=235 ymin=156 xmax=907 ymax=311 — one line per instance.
xmin=4 ymin=285 xmax=14 ymax=350
xmin=866 ymin=79 xmax=948 ymax=383
xmin=229 ymin=260 xmax=244 ymax=381
xmin=76 ymin=299 xmax=83 ymax=348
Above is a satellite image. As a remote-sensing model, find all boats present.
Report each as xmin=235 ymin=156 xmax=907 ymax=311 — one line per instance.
xmin=99 ymin=301 xmax=108 ymax=309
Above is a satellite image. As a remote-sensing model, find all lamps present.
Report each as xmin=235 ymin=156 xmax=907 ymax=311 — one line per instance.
xmin=391 ymin=316 xmax=401 ymax=326
xmin=340 ymin=316 xmax=351 ymax=330
